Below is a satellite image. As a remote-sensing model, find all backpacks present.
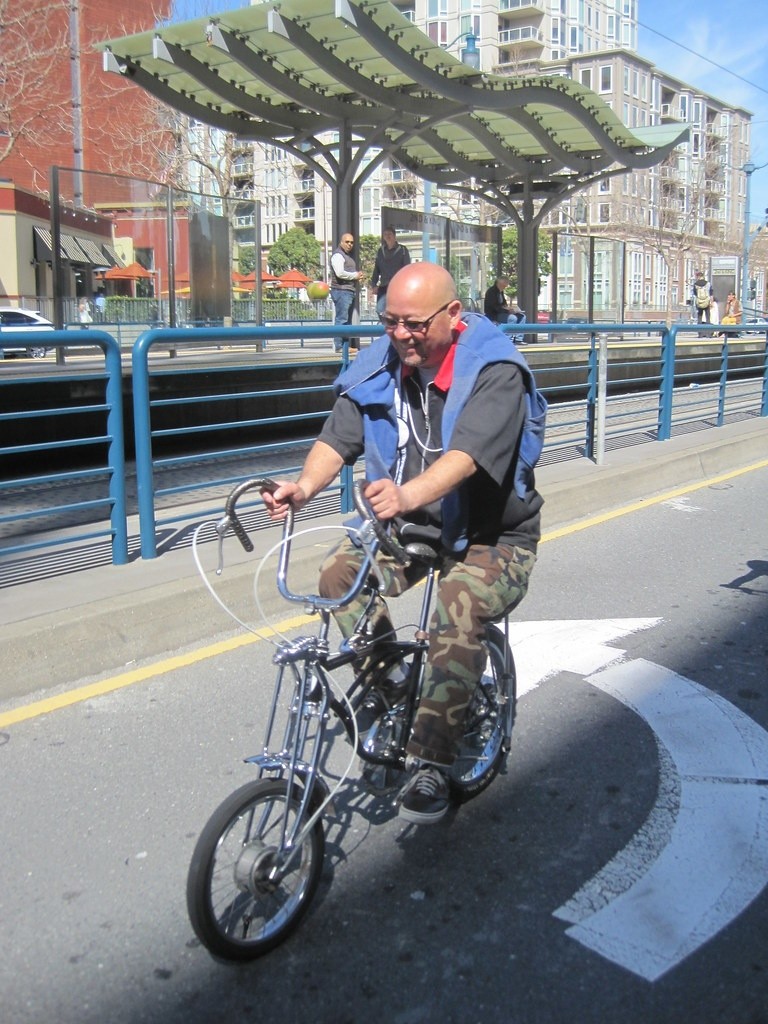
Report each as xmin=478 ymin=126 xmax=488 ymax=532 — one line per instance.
xmin=694 ymin=282 xmax=710 ymax=309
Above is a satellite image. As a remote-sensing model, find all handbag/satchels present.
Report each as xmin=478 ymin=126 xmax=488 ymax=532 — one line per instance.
xmin=720 ymin=316 xmax=736 ymax=325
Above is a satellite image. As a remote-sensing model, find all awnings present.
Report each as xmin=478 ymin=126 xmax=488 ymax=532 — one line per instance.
xmin=35 ymin=228 xmax=126 ymax=267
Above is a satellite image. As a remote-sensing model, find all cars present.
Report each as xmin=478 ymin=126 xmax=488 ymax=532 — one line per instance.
xmin=562 ymin=317 xmax=590 ymax=338
xmin=0 ymin=308 xmax=56 ymax=361
xmin=537 ymin=307 xmax=554 ymax=325
xmin=744 ymin=317 xmax=768 ymax=335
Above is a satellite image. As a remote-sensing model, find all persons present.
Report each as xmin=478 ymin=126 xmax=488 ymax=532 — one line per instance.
xmin=714 ymin=290 xmax=744 ymax=337
xmin=694 ymin=272 xmax=713 ymax=338
xmin=329 ymin=233 xmax=363 ymax=353
xmin=96 ymin=293 xmax=106 ymax=323
xmin=78 ymin=297 xmax=93 ymax=328
xmin=368 ymin=227 xmax=411 ymax=324
xmin=258 ymin=260 xmax=548 ymax=822
xmin=484 ymin=276 xmax=529 ymax=346
xmin=148 ymin=282 xmax=153 ymax=297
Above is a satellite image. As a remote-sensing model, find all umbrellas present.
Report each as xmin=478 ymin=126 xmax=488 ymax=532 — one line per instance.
xmin=160 ymin=267 xmax=312 ymax=300
xmin=115 ymin=261 xmax=155 ymax=298
xmin=95 ymin=264 xmax=140 ymax=293
xmin=278 ymin=269 xmax=313 ymax=299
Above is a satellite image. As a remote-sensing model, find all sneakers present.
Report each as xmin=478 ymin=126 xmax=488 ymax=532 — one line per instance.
xmin=357 ymin=662 xmax=412 ymax=741
xmin=399 ymin=762 xmax=450 ymax=823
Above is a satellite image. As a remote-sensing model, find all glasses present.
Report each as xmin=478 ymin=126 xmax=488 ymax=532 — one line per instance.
xmin=341 ymin=240 xmax=354 ymax=244
xmin=379 ymin=301 xmax=453 ymax=333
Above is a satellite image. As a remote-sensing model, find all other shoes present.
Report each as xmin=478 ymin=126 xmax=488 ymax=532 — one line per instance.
xmin=336 ymin=347 xmax=358 ymax=355
xmin=515 ymin=340 xmax=527 ymax=345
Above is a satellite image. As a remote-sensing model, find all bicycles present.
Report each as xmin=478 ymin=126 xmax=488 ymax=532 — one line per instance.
xmin=185 ymin=478 xmax=518 ymax=966
